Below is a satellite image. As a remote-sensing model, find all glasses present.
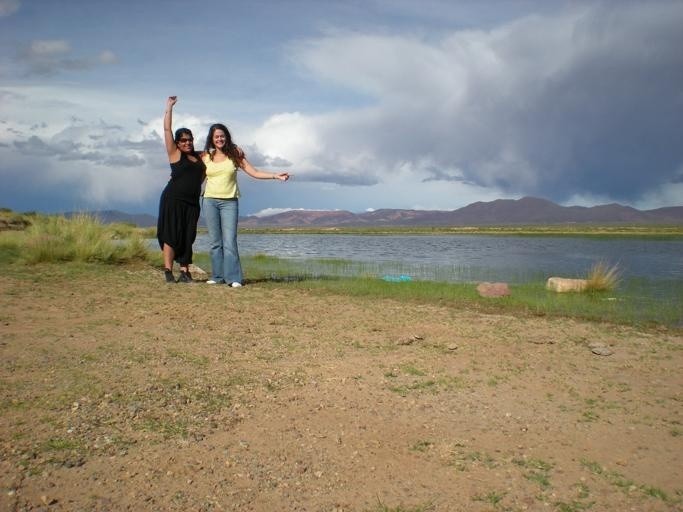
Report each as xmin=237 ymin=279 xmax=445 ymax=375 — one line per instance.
xmin=177 ymin=137 xmax=193 ymax=143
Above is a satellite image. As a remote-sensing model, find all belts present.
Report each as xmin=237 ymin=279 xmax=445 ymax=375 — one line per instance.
xmin=212 ymin=198 xmax=238 ymax=201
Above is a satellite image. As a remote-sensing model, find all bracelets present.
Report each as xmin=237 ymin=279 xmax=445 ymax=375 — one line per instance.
xmin=273 ymin=173 xmax=276 ymax=179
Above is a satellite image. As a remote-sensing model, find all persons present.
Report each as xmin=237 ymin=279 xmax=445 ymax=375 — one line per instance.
xmin=155 ymin=94 xmax=244 ymax=283
xmin=193 ymin=123 xmax=294 ymax=288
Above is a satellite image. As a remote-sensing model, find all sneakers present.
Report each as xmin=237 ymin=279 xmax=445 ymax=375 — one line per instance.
xmin=206 ymin=280 xmax=224 ymax=284
xmin=165 ymin=270 xmax=177 ymax=283
xmin=229 ymin=281 xmax=242 ymax=287
xmin=180 ymin=270 xmax=195 ymax=283
